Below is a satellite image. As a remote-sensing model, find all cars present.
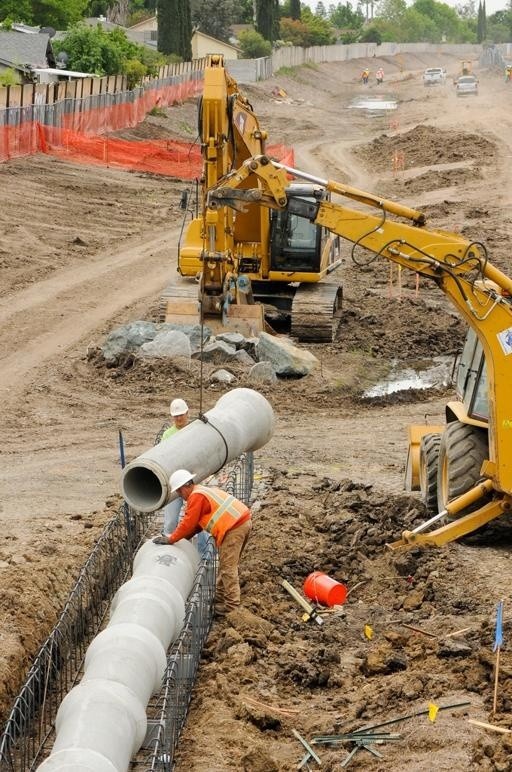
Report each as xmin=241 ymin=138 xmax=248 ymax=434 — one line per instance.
xmin=454 ymin=75 xmax=480 ymax=99
xmin=422 ymin=68 xmax=448 ymax=87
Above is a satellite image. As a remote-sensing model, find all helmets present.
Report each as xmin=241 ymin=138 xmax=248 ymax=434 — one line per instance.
xmin=169 ymin=398 xmax=189 ymax=417
xmin=168 ymin=469 xmax=197 ymax=493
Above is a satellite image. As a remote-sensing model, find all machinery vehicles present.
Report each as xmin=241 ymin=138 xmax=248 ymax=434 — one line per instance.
xmin=164 ymin=52 xmax=342 ymax=343
xmin=205 ymin=153 xmax=512 ymax=558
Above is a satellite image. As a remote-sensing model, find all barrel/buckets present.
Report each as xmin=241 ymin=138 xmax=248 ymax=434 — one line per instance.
xmin=303 ymin=569 xmax=347 ymax=608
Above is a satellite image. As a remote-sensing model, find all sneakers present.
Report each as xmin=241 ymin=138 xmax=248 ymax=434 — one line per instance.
xmin=212 ymin=604 xmax=236 ymax=616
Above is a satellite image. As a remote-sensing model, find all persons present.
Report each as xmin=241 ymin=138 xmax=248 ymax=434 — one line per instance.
xmin=375 ymin=68 xmax=384 ymax=85
xmin=362 ymin=67 xmax=369 ymax=84
xmin=161 ymin=399 xmax=209 ymax=552
xmin=153 ymin=470 xmax=252 ymax=616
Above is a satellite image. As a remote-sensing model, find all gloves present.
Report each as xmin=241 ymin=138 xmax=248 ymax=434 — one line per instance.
xmin=152 ymin=533 xmax=169 ymax=544
